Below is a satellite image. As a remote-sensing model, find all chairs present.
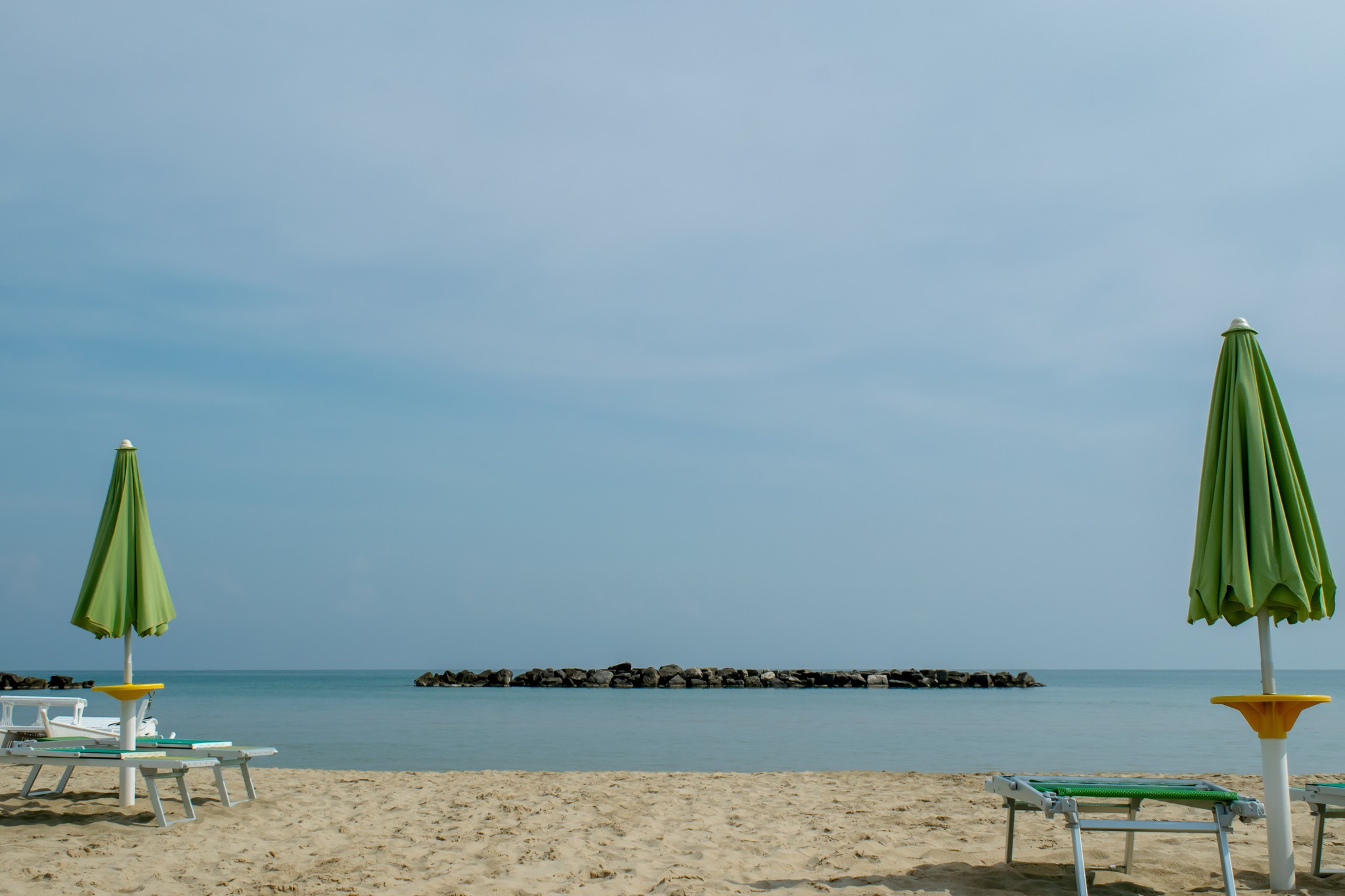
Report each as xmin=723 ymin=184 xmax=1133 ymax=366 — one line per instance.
xmin=1282 ymin=782 xmax=1345 ymax=879
xmin=978 ymin=772 xmax=1268 ymax=896
xmin=0 ymin=733 xmax=278 ymax=826
